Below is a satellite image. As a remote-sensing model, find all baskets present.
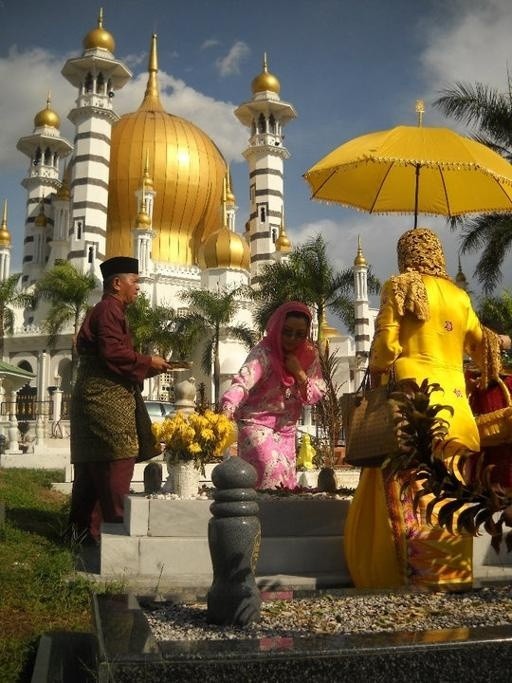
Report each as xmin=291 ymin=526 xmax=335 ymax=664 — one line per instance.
xmin=469 ymin=374 xmax=512 ymax=447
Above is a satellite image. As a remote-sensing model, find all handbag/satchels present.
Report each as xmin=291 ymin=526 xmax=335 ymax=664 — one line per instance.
xmin=339 ymin=365 xmax=416 ymax=469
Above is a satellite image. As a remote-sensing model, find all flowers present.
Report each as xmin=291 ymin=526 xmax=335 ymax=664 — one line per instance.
xmin=151 ymin=407 xmax=238 ymax=465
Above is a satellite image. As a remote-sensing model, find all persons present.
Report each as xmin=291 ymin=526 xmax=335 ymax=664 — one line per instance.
xmin=222 ymin=299 xmax=319 ymax=491
xmin=364 ymin=227 xmax=511 ymax=469
xmin=67 ymin=256 xmax=175 ymax=547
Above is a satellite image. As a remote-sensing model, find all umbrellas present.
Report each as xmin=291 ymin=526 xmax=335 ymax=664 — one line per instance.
xmin=303 ymin=95 xmax=512 ymax=233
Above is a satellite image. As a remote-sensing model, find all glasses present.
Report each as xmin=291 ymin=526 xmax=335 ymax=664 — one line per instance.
xmin=282 ymin=328 xmax=308 ymax=337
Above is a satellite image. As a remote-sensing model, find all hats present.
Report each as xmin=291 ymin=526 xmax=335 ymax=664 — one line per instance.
xmin=99 ymin=257 xmax=138 ymax=277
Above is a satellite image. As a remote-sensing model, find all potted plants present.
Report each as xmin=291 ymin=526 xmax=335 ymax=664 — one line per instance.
xmin=317 ymin=339 xmax=350 ymax=465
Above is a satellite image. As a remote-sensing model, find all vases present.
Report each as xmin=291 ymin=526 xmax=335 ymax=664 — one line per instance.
xmin=166 ymin=448 xmax=200 ymax=496
